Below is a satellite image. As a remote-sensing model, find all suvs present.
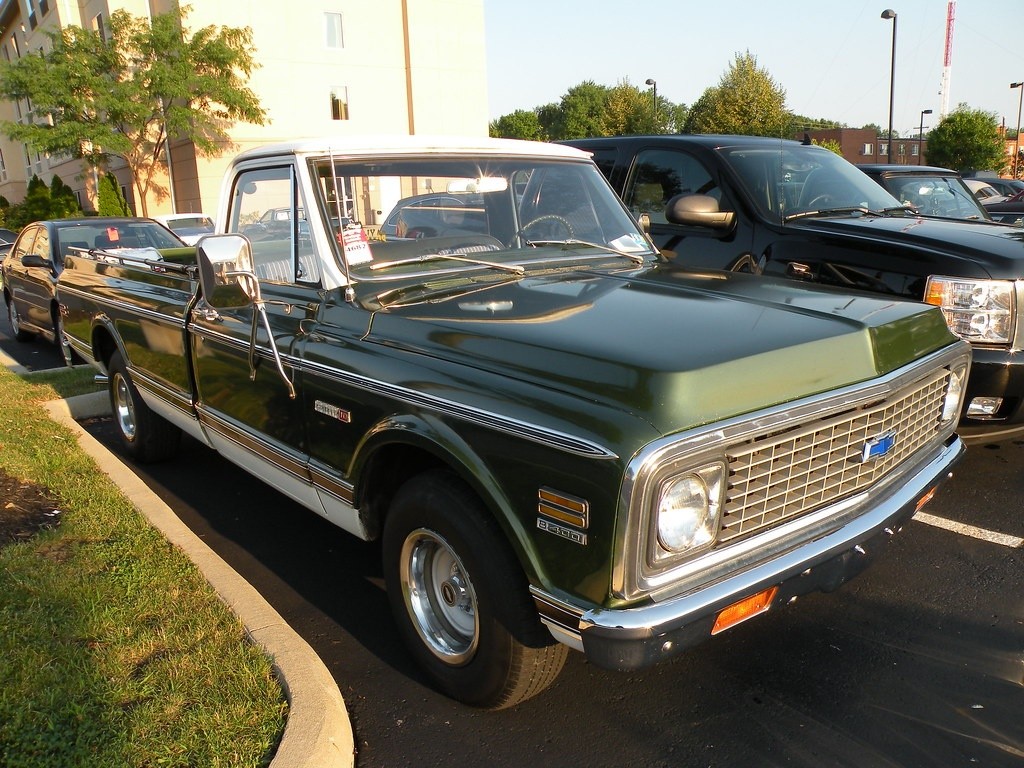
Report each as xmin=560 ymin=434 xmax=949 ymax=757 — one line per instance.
xmin=377 ymin=190 xmax=524 ymax=241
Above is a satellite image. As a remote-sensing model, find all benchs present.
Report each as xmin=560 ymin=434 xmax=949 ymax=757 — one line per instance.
xmin=349 ymin=234 xmax=505 ymax=270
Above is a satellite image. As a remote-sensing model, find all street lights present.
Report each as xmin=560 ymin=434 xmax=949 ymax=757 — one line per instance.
xmin=645 ymin=79 xmax=657 ymax=117
xmin=918 ymin=110 xmax=932 ymax=163
xmin=1010 ymin=82 xmax=1024 ymax=180
xmin=881 ymin=9 xmax=898 ymax=164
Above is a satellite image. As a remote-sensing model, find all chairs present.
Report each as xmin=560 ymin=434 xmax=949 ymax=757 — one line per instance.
xmin=660 ymin=167 xmax=682 ymax=203
xmin=94 ymin=235 xmax=120 ymax=249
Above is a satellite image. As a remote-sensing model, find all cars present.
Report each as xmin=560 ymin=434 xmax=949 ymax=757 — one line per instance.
xmin=961 ymin=177 xmax=1024 ymax=229
xmin=0 ymin=227 xmax=18 ymax=264
xmin=1 ymin=217 xmax=189 ymax=342
xmin=154 ymin=213 xmax=215 ymax=245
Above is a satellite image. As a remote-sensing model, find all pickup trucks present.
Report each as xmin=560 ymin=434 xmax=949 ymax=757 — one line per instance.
xmin=522 ymin=137 xmax=1024 ymax=447
xmin=396 ymin=165 xmax=1024 ymax=255
xmin=55 ymin=135 xmax=972 ymax=710
xmin=239 ymin=207 xmax=349 ymax=242
